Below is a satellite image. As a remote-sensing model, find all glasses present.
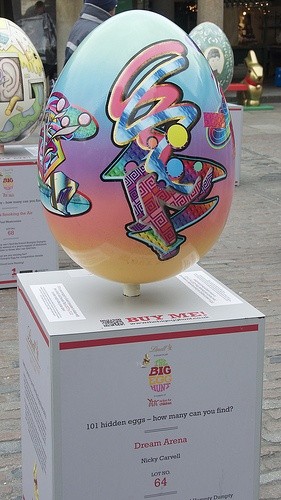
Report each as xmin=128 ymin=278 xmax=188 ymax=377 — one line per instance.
xmin=113 ymin=2 xmax=120 ymax=8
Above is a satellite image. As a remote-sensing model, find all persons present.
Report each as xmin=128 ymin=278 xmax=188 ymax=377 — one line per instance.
xmin=207 ymin=48 xmax=220 ymax=80
xmin=31 ymin=1 xmax=56 ymax=67
xmin=64 ymin=0 xmax=119 ymax=69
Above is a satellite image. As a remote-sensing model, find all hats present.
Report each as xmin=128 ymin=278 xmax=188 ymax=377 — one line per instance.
xmin=84 ymin=0 xmax=117 ymax=11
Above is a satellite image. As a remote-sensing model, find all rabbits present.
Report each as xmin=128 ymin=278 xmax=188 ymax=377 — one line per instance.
xmin=236 ymin=49 xmax=263 ymax=108
